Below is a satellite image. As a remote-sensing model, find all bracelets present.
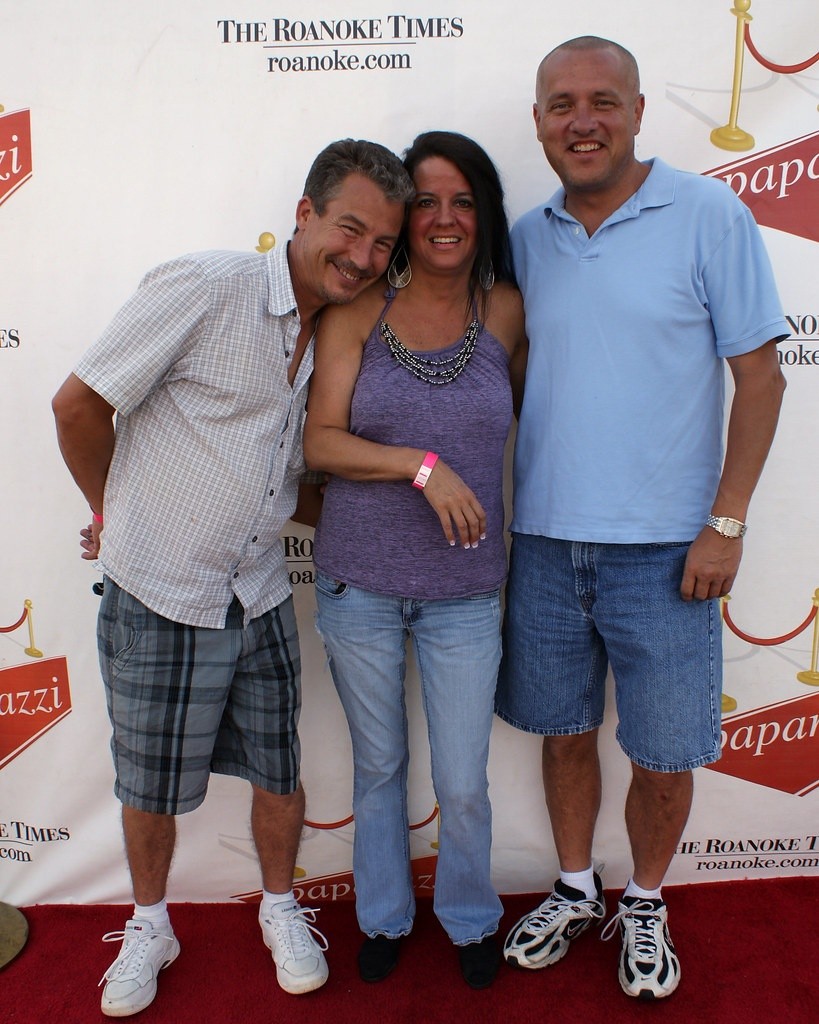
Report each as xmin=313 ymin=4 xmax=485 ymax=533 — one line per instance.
xmin=412 ymin=452 xmax=439 ymax=490
xmin=94 ymin=512 xmax=103 ymax=522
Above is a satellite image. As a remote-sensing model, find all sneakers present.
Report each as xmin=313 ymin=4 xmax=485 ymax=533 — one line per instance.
xmin=600 ymin=896 xmax=682 ymax=1000
xmin=503 ymin=857 xmax=606 ymax=969
xmin=258 ymin=900 xmax=329 ymax=995
xmin=98 ymin=910 xmax=181 ymax=1017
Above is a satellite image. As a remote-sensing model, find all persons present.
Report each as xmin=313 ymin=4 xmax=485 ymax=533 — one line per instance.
xmin=303 ymin=133 xmax=527 ymax=988
xmin=50 ymin=137 xmax=417 ymax=1017
xmin=495 ymin=35 xmax=791 ymax=1001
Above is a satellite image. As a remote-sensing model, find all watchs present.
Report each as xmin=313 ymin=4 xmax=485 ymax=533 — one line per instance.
xmin=705 ymin=514 xmax=747 ymax=538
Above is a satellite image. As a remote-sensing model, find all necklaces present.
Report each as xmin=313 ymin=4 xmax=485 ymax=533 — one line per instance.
xmin=379 ymin=319 xmax=480 ymax=385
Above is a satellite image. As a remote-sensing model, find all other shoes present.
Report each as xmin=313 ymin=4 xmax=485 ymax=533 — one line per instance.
xmin=358 ymin=936 xmax=401 ymax=982
xmin=459 ymin=935 xmax=498 ymax=989
